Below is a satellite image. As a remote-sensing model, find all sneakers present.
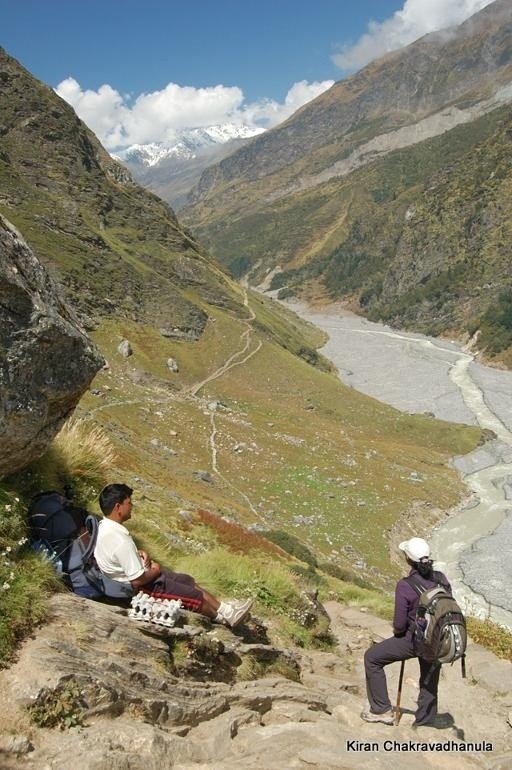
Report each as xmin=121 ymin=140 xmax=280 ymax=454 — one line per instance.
xmin=360 ymin=706 xmax=396 ymax=725
xmin=223 ymin=596 xmax=255 ymax=629
xmin=215 ymin=612 xmax=253 ymax=629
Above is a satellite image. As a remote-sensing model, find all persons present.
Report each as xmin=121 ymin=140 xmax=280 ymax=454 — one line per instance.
xmin=361 ymin=537 xmax=453 ymax=727
xmin=91 ymin=484 xmax=254 ymax=628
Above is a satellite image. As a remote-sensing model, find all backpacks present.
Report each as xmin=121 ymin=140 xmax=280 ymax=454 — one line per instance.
xmin=27 ymin=489 xmax=90 ymax=569
xmin=400 ymin=570 xmax=468 ymax=666
xmin=53 ymin=515 xmax=135 ymax=602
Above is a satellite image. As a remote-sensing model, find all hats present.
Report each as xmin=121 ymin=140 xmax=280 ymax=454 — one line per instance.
xmin=397 ymin=536 xmax=432 ymax=564
